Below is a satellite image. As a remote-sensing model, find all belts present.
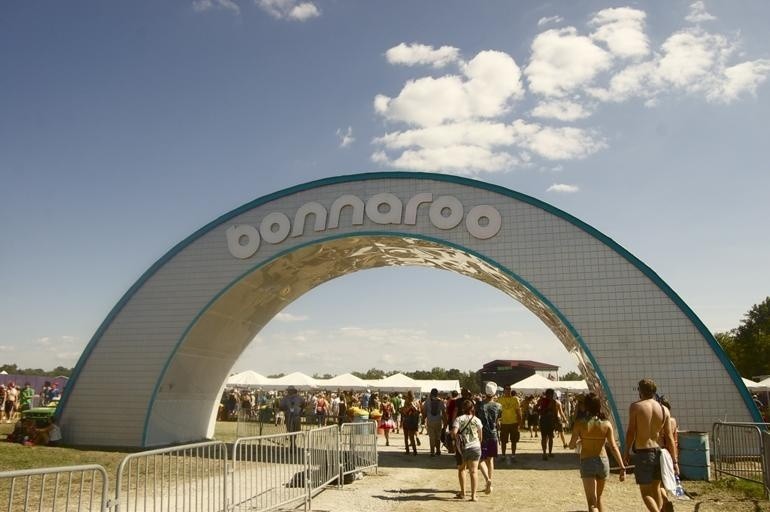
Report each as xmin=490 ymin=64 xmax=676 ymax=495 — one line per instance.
xmin=634 ymin=445 xmax=661 ymax=453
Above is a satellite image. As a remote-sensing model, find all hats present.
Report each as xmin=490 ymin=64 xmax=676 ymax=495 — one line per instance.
xmin=484 ymin=379 xmax=498 ymax=397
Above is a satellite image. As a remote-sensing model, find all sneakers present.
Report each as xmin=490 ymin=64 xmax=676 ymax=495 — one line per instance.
xmin=484 ymin=478 xmax=492 ymax=495
xmin=499 ymin=454 xmax=518 ymax=464
xmin=543 ymin=453 xmax=547 ymax=460
xmin=563 ymin=443 xmax=569 ymax=449
xmin=530 ymin=434 xmax=562 ymax=438
xmin=384 ymin=428 xmax=456 ymax=457
xmin=548 ymin=453 xmax=555 ymax=457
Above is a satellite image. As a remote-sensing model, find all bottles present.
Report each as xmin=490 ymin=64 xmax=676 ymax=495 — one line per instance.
xmin=675 ymin=474 xmax=684 ymax=496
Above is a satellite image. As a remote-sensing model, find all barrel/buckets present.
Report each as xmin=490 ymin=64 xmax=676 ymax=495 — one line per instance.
xmin=676 ymin=430 xmax=711 ymax=481
xmin=353 ymin=415 xmax=369 ymax=435
xmin=370 ymin=414 xmax=384 ymax=435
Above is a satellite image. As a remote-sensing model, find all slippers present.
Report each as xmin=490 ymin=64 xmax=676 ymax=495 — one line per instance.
xmin=471 ymin=494 xmax=480 ymax=502
xmin=455 ymin=491 xmax=467 ymax=499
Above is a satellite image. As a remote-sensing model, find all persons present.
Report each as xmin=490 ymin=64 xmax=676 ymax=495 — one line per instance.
xmin=217 ymin=384 xmax=348 ymax=441
xmin=344 ymin=388 xmax=430 ymax=456
xmin=563 ymin=376 xmax=683 ymax=511
xmin=1 ymin=380 xmax=62 ymax=423
xmin=425 ymin=381 xmax=568 ymax=501
